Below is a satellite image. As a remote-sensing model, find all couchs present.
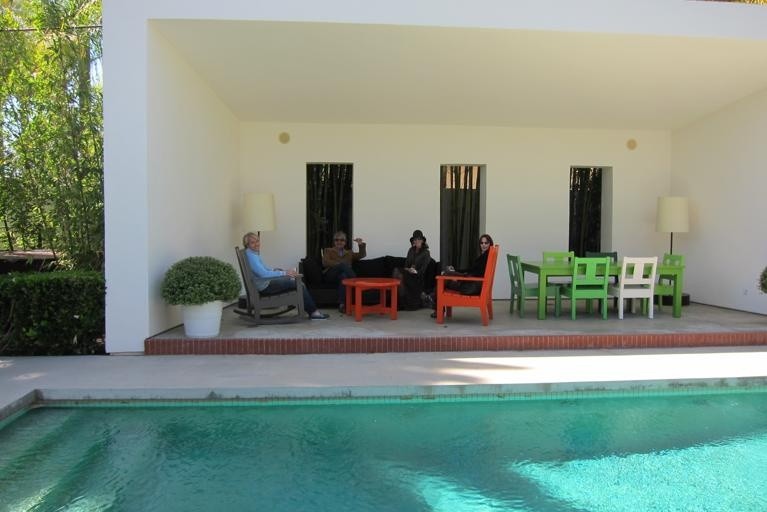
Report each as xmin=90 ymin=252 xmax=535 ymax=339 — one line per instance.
xmin=298 ymin=255 xmax=441 ymax=305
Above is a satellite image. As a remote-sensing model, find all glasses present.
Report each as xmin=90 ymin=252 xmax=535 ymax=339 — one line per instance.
xmin=335 ymin=239 xmax=344 ymax=242
xmin=481 ymin=242 xmax=489 ymax=244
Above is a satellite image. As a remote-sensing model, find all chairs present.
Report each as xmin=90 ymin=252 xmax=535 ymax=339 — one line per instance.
xmin=435 ymin=244 xmax=499 ymax=326
xmin=233 ymin=245 xmax=311 ymax=324
xmin=505 ymin=251 xmax=685 ymax=319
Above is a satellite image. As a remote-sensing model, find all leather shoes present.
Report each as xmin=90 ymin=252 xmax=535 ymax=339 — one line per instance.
xmin=311 ymin=313 xmax=329 ymax=318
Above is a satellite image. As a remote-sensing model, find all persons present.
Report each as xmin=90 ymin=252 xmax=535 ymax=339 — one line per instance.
xmin=399 ymin=231 xmax=431 ymax=312
xmin=424 ymin=235 xmax=493 ymax=317
xmin=324 ymin=230 xmax=368 ymax=313
xmin=242 ymin=232 xmax=330 ymax=321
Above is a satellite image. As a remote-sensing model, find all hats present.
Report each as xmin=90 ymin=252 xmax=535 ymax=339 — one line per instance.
xmin=410 ymin=230 xmax=426 ymax=242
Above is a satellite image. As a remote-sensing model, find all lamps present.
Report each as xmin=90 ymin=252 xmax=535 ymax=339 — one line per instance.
xmin=648 ymin=196 xmax=689 ymax=305
xmin=237 ymin=193 xmax=277 ymax=310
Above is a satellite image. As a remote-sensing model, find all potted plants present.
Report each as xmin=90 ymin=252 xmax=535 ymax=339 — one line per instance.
xmin=160 ymin=256 xmax=242 ymax=339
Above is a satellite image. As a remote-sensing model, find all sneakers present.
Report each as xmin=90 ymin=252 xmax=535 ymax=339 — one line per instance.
xmin=421 ymin=291 xmax=437 ymax=318
xmin=339 ymin=304 xmax=345 ymax=313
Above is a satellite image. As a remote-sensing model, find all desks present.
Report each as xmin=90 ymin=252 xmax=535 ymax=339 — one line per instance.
xmin=341 ymin=277 xmax=400 ymax=321
